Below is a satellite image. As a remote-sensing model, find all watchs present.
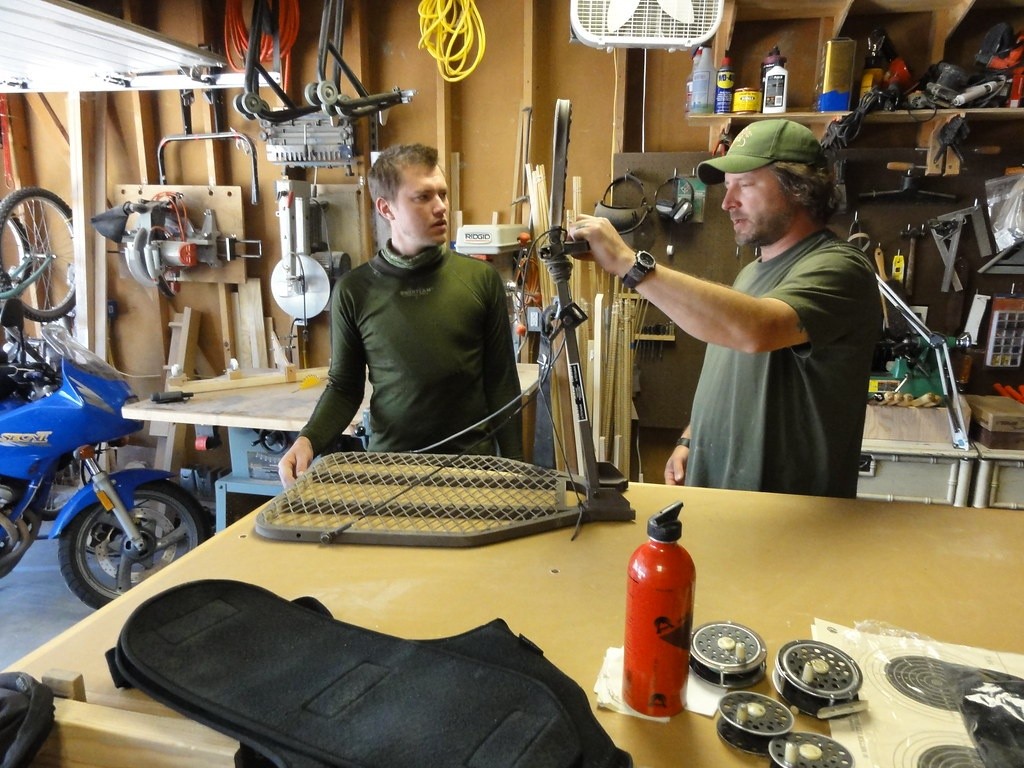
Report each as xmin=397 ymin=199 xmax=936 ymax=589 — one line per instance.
xmin=622 ymin=250 xmax=656 ymax=288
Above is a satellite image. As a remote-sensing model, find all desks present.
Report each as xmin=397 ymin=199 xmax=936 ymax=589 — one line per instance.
xmin=120 ymin=363 xmax=541 ymax=533
xmin=1 ymin=455 xmax=1023 ymax=768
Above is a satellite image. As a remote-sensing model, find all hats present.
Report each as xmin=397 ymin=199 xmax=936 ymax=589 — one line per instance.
xmin=695 ymin=120 xmax=826 ymax=185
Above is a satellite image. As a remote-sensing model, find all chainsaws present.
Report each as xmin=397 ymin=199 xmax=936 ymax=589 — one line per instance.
xmin=270 ymin=180 xmax=351 ymax=327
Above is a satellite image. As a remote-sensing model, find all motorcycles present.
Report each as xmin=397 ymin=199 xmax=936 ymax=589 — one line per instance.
xmin=1 ymin=326 xmax=211 ymax=610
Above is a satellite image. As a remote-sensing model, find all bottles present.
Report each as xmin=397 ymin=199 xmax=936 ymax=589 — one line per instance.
xmin=685 ymin=45 xmax=715 ymax=117
xmin=714 ymin=49 xmax=733 ymax=115
xmin=760 ymin=45 xmax=789 ymax=116
xmin=622 ymin=500 xmax=696 ymax=720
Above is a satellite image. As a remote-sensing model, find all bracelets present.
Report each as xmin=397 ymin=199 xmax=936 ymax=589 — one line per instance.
xmin=675 ymin=437 xmax=690 ymax=447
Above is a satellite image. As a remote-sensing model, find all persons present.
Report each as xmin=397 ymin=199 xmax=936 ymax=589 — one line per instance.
xmin=279 ymin=143 xmax=522 ymax=491
xmin=569 ymin=119 xmax=876 ymax=498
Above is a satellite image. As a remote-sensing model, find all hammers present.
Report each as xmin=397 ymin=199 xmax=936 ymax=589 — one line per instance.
xmin=900 ymin=229 xmax=927 ymax=297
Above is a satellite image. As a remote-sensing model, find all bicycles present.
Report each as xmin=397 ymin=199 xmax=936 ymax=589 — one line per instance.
xmin=0 ymin=187 xmax=101 ymax=522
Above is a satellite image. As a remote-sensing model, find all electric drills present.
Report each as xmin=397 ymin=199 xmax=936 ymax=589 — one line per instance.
xmin=950 ymin=77 xmax=1011 ymax=109
